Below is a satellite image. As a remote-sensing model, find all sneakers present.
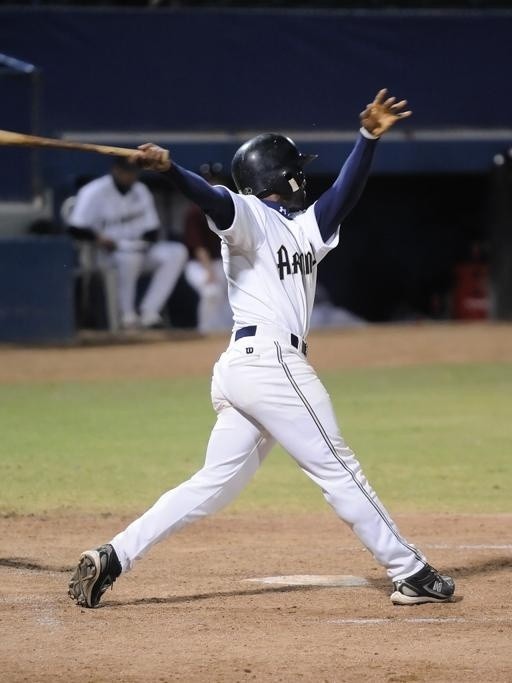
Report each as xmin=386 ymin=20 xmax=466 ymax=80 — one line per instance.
xmin=392 ymin=563 xmax=456 ymax=607
xmin=67 ymin=542 xmax=121 ymax=607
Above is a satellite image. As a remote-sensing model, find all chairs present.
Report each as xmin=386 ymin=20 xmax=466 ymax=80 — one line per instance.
xmin=60 ymin=196 xmax=171 ymax=334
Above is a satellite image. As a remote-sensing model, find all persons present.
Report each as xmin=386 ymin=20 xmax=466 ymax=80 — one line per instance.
xmin=69 ymin=86 xmax=456 ymax=607
xmin=63 ymin=153 xmax=190 ymax=329
xmin=176 ymin=165 xmax=230 ymax=331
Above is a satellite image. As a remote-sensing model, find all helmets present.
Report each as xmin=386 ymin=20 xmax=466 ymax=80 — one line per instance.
xmin=230 ymin=131 xmax=320 ymax=211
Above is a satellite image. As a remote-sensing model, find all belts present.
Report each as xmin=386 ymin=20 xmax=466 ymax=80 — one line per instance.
xmin=235 ymin=325 xmax=308 ymax=356
